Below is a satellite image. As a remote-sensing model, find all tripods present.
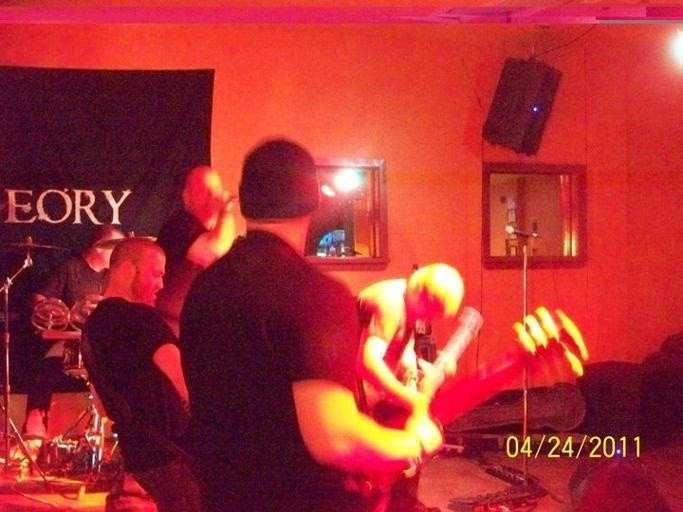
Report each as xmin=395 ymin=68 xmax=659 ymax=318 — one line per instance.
xmin=0 ymin=258 xmax=55 ymax=495
xmin=61 ymin=385 xmax=119 ymax=491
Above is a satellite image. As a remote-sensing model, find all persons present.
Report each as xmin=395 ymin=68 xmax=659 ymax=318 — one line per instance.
xmin=19 ymin=138 xmax=465 ymax=512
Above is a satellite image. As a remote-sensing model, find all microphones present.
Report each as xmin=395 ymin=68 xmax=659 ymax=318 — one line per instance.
xmin=505 ymin=226 xmax=541 ymax=237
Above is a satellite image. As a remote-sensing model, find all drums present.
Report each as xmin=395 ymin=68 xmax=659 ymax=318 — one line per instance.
xmin=62 ymin=338 xmax=90 ymax=379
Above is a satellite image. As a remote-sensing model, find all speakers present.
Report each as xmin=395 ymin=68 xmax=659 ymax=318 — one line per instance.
xmin=482 ymin=57 xmax=562 ymax=156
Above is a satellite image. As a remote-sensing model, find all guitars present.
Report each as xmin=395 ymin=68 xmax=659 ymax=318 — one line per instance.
xmin=368 ymin=307 xmax=483 ymax=464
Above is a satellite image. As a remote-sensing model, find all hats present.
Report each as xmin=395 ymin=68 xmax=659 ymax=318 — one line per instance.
xmin=238 ymin=140 xmax=320 ymax=218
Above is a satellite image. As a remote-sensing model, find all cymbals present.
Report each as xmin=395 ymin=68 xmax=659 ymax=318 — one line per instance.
xmin=95 ymin=236 xmax=157 ymax=249
xmin=1 ymin=242 xmax=70 ymax=255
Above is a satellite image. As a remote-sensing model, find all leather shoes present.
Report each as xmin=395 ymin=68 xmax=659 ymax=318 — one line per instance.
xmin=21 ymin=408 xmax=47 ymax=438
